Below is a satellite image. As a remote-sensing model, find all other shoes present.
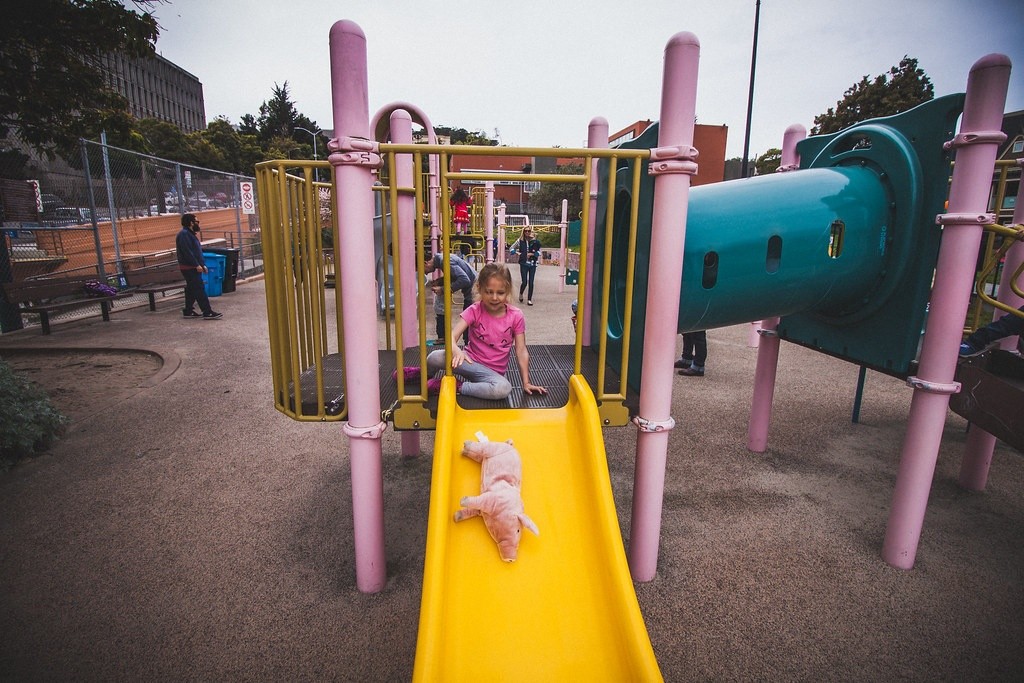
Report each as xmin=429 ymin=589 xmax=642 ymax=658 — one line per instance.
xmin=433 ymin=340 xmax=445 ymax=345
xmin=519 ymin=293 xmax=523 ymax=303
xmin=527 ymin=300 xmax=533 ymax=305
xmin=182 ymin=308 xmax=223 ymax=319
xmin=674 ymin=360 xmax=704 ymax=377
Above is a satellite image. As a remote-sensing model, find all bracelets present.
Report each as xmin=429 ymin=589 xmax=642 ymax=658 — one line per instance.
xmin=524 ymin=382 xmax=530 ymax=386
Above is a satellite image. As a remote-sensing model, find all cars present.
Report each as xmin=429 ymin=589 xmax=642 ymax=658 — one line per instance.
xmin=42 ymin=207 xmax=110 ymax=227
xmin=41 ymin=194 xmax=67 ymax=208
xmin=142 ymin=191 xmax=237 ymax=217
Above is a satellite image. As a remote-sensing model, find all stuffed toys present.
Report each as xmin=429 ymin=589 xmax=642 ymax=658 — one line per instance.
xmin=453 ymin=430 xmax=538 ymax=563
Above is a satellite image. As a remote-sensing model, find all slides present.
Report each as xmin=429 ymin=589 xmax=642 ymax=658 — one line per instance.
xmin=374 ymin=183 xmax=429 ymax=316
xmin=410 ymin=374 xmax=667 ymax=683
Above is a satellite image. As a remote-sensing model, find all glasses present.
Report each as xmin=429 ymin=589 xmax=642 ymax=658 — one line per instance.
xmin=524 ymin=230 xmax=531 ymax=233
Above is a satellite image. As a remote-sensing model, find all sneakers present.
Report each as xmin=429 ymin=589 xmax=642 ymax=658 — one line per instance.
xmin=393 ymin=367 xmax=421 ymax=380
xmin=428 ymin=378 xmax=462 ymax=392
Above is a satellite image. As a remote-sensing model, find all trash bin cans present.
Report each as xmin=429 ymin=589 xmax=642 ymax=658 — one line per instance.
xmin=202 ymin=252 xmax=226 ymax=296
xmin=202 ymin=247 xmax=239 ymax=294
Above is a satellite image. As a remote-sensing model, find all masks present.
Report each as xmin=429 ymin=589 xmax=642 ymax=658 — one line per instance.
xmin=194 ymin=221 xmax=199 ymax=224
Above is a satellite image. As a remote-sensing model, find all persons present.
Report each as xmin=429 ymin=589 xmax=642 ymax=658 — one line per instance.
xmin=416 ymin=226 xmax=541 ymax=345
xmin=674 ymin=330 xmax=707 ymax=376
xmin=957 ymin=305 xmax=1024 ymax=358
xmin=176 ymin=214 xmax=223 ymax=320
xmin=393 ymin=262 xmax=547 ymax=400
xmin=448 ymin=187 xmax=475 ymax=234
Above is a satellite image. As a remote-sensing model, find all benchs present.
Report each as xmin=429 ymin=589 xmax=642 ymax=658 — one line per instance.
xmin=18 ymin=273 xmax=132 ymax=335
xmin=124 ymin=260 xmax=189 ymax=311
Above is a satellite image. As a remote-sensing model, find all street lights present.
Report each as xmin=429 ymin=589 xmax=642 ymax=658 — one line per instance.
xmin=293 ymin=126 xmax=327 ymax=182
